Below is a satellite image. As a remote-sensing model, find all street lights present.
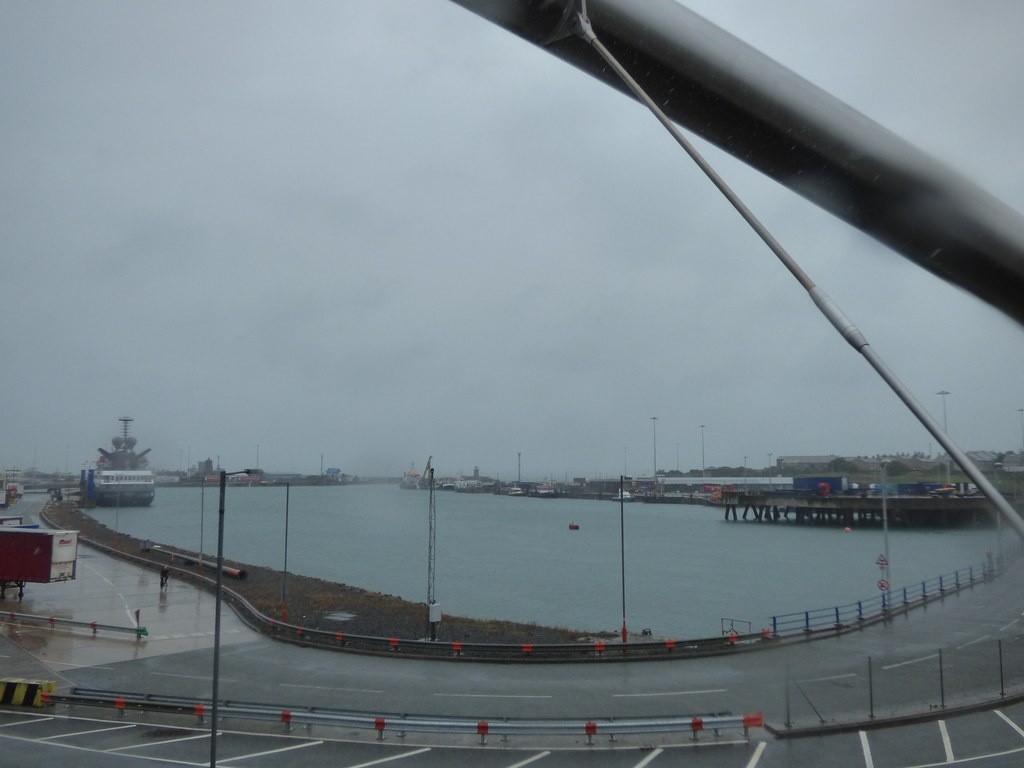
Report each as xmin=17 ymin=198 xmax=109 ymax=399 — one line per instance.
xmin=879 ymin=458 xmax=892 ymax=608
xmin=208 ymin=468 xmax=264 ymax=768
xmin=261 ymin=480 xmax=289 ymax=609
xmin=994 ymin=462 xmax=1005 ymax=575
xmin=200 ymin=476 xmax=207 ymax=576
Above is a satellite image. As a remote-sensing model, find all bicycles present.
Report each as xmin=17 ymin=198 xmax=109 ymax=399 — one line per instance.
xmin=160 ymin=574 xmax=167 ymax=591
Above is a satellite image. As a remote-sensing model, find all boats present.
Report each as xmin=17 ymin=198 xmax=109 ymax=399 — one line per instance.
xmin=401 ymin=462 xmax=420 ymax=490
xmin=569 ymin=524 xmax=579 ymax=529
xmin=612 ymin=488 xmax=635 ymax=501
xmin=92 ymin=415 xmax=154 ymax=506
xmin=508 ymin=487 xmax=523 ymax=495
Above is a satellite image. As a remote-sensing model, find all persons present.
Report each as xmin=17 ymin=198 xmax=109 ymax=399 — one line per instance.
xmin=160 ymin=566 xmax=168 ymax=588
